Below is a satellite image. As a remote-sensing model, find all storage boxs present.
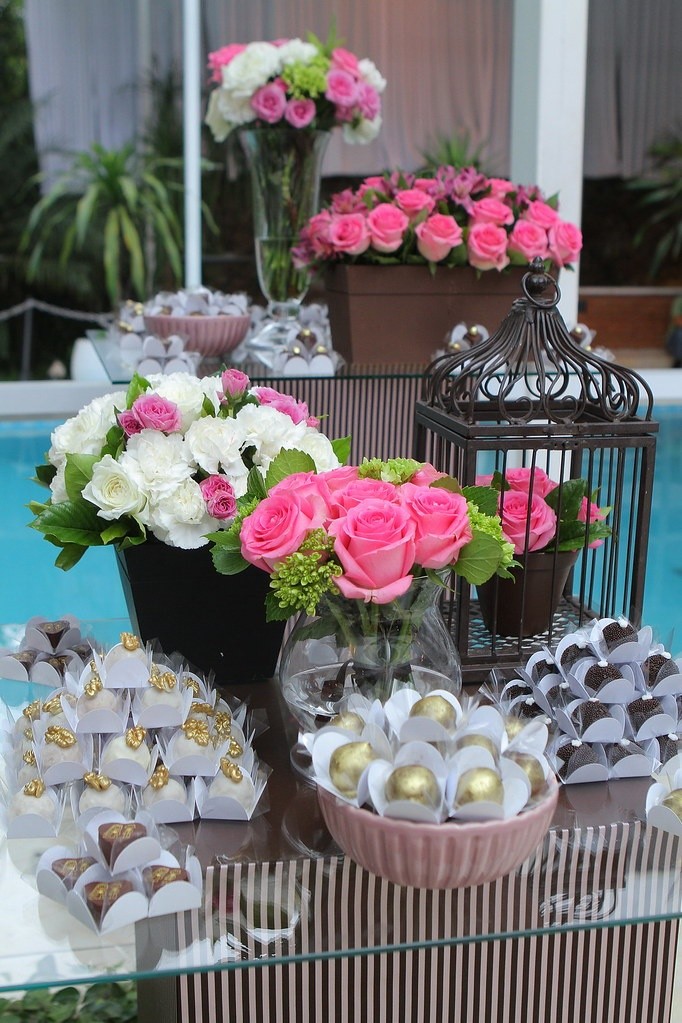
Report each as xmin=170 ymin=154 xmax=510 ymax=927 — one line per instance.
xmin=308 ymin=756 xmax=560 ymax=895
xmin=139 ymin=309 xmax=250 ymax=355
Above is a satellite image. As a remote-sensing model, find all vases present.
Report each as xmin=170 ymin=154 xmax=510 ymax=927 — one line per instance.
xmin=326 ymin=262 xmax=558 ymax=373
xmin=111 ymin=540 xmax=292 ymax=684
xmin=233 ymin=129 xmax=330 ymax=320
xmin=475 ymin=550 xmax=578 ymax=639
xmin=279 ymin=566 xmax=463 ymax=743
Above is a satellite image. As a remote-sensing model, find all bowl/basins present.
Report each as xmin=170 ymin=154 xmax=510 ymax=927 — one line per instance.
xmin=318 ymin=739 xmax=558 ymax=892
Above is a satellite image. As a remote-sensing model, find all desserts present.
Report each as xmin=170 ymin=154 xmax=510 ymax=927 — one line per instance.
xmin=7 ymin=621 xmax=252 ymax=927
xmin=320 ymin=694 xmax=545 ymax=806
xmin=502 ymin=621 xmax=682 ymax=823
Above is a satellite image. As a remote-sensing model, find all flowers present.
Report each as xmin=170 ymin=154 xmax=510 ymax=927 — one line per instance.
xmin=463 ymin=466 xmax=612 ymax=553
xmin=204 ymin=36 xmax=388 ymax=142
xmin=292 ymin=169 xmax=583 ymax=272
xmin=239 ymin=458 xmax=514 ymax=603
xmin=46 ymin=371 xmax=343 ymax=551
